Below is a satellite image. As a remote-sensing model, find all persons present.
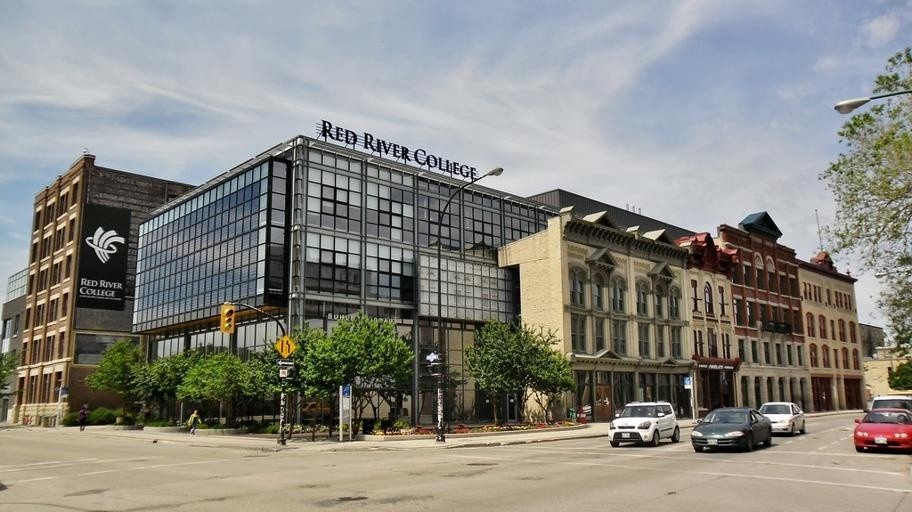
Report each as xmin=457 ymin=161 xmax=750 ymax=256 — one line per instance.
xmin=187 ymin=408 xmax=203 ymax=436
xmin=893 ymin=413 xmax=906 ymax=423
xmin=79 ymin=403 xmax=89 ymax=432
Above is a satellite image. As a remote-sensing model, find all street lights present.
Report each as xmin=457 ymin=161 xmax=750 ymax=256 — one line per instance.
xmin=435 ymin=168 xmax=504 ymax=443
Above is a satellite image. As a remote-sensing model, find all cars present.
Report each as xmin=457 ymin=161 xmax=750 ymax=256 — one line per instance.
xmin=854 ymin=396 xmax=912 ymax=452
xmin=691 ymin=402 xmax=806 ymax=452
xmin=608 ymin=401 xmax=680 ymax=447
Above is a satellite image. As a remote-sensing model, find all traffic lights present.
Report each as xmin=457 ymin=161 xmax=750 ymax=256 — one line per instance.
xmin=220 ymin=304 xmax=235 ymax=334
